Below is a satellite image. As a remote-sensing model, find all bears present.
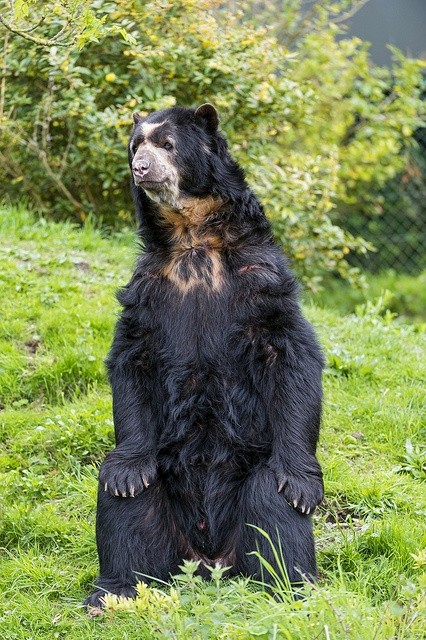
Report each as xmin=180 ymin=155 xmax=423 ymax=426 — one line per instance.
xmin=82 ymin=102 xmax=325 ymax=614
